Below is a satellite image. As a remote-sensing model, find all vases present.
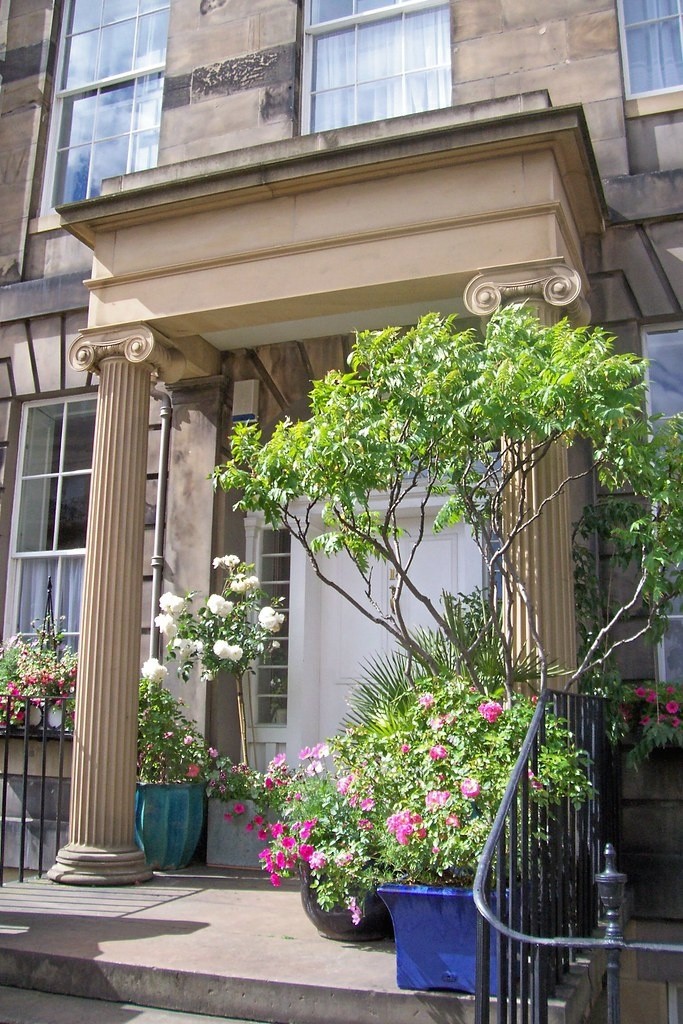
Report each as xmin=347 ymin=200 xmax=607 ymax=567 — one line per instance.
xmin=300 ymin=860 xmax=394 ymax=941
xmin=207 ymin=796 xmax=294 ymax=869
xmin=134 ymin=781 xmax=209 ymax=871
xmin=377 ymin=881 xmax=511 ymax=997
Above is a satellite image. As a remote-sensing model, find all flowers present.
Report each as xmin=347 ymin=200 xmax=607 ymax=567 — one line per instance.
xmin=160 ymin=552 xmax=599 ymax=924
xmin=0 ymin=615 xmax=79 ymax=728
xmin=136 ymin=678 xmax=219 ymax=784
xmin=616 ymin=680 xmax=683 ymax=773
